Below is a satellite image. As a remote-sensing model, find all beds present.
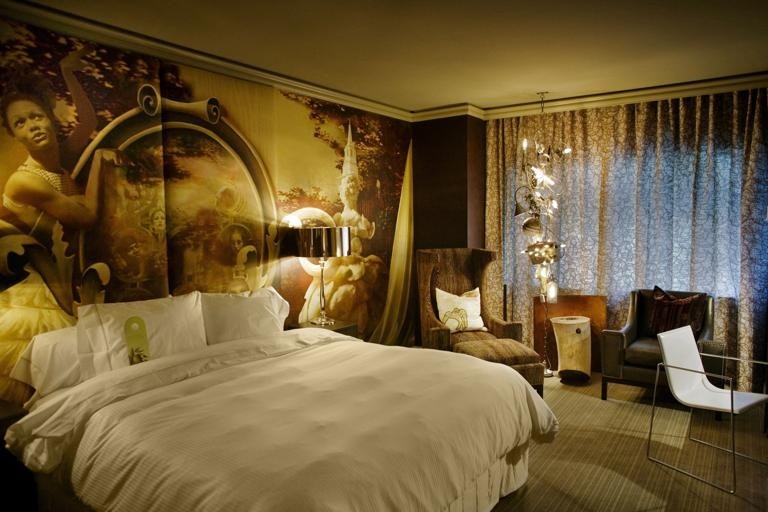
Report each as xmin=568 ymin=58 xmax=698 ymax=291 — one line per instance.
xmin=5 ymin=329 xmax=558 ymax=512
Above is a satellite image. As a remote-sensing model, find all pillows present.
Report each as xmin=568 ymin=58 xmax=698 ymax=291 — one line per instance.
xmin=436 ymin=287 xmax=488 ymax=334
xmin=202 ymin=287 xmax=290 ymax=345
xmin=648 ymin=284 xmax=707 ymax=338
xmin=78 ymin=291 xmax=207 ymax=383
xmin=9 ymin=325 xmax=78 ymax=398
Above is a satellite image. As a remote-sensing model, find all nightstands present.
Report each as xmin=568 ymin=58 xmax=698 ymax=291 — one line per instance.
xmin=287 ymin=316 xmax=358 ymax=339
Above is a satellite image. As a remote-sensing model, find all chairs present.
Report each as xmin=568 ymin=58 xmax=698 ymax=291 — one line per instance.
xmin=417 ymin=248 xmax=545 ymax=401
xmin=646 ymin=324 xmax=768 ymax=495
xmin=599 ymin=289 xmax=726 ymax=421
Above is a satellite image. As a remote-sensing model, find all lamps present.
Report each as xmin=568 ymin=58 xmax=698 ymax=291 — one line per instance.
xmin=513 ymin=89 xmax=572 ymax=377
xmin=279 ymin=226 xmax=352 ymax=326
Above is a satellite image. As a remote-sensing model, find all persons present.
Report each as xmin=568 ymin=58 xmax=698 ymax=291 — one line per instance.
xmin=2 ymin=50 xmax=136 ymax=239
xmin=111 ymin=224 xmax=152 ymax=281
xmin=147 ymin=203 xmax=167 ymax=251
xmin=211 ymin=221 xmax=254 ymax=269
